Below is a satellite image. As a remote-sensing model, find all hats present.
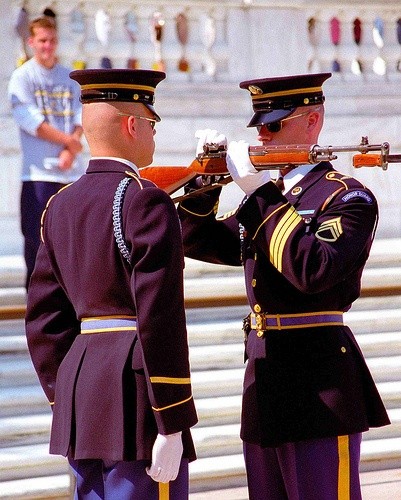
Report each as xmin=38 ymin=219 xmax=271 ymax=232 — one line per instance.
xmin=69 ymin=68 xmax=166 ymax=122
xmin=239 ymin=73 xmax=332 ymax=127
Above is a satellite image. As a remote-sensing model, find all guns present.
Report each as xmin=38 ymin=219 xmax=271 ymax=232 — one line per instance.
xmin=138 ymin=135 xmax=401 ymax=205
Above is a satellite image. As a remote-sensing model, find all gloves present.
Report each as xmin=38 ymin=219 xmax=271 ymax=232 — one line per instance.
xmin=226 ymin=140 xmax=271 ymax=196
xmin=145 ymin=432 xmax=184 ymax=483
xmin=194 ymin=128 xmax=227 ymax=183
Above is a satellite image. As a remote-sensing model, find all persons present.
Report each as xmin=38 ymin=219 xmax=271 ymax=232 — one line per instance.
xmin=24 ymin=70 xmax=200 ymax=500
xmin=11 ymin=0 xmax=401 ymax=76
xmin=179 ymin=72 xmax=392 ymax=500
xmin=7 ymin=16 xmax=88 ymax=299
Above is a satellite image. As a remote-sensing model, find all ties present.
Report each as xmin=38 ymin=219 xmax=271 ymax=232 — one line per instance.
xmin=275 ymin=177 xmax=284 ymax=191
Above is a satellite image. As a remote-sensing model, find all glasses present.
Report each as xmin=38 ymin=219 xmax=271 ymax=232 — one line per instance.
xmin=116 ymin=113 xmax=155 ymax=129
xmin=257 ymin=106 xmax=320 ymax=134
